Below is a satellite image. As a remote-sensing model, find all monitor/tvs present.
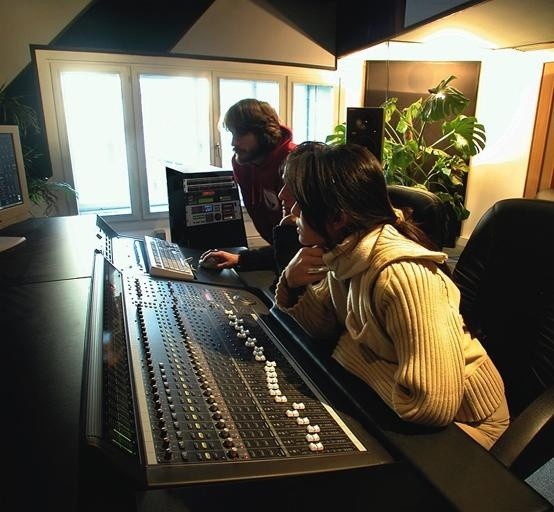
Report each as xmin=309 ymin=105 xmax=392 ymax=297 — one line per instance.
xmin=0 ymin=125 xmax=31 ymax=252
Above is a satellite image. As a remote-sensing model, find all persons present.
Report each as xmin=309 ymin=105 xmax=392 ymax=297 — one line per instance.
xmin=273 ymin=142 xmax=512 ymax=451
xmin=221 ymin=98 xmax=300 ymax=246
xmin=198 ymin=139 xmax=331 ymax=277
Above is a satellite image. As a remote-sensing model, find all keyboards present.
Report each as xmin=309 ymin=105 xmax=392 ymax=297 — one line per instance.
xmin=143 ymin=235 xmax=194 ymax=280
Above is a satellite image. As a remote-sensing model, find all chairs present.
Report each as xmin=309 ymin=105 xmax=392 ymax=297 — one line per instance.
xmin=387 ymin=182 xmax=553 ymax=483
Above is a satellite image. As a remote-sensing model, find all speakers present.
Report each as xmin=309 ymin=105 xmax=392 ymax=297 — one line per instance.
xmin=166 ymin=166 xmax=248 ymax=248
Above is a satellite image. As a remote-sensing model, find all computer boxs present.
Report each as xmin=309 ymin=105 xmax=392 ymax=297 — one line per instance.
xmin=347 ymin=107 xmax=385 ymax=164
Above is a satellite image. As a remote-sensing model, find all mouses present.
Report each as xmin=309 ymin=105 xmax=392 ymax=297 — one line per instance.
xmin=198 ymin=255 xmax=223 ymax=269
